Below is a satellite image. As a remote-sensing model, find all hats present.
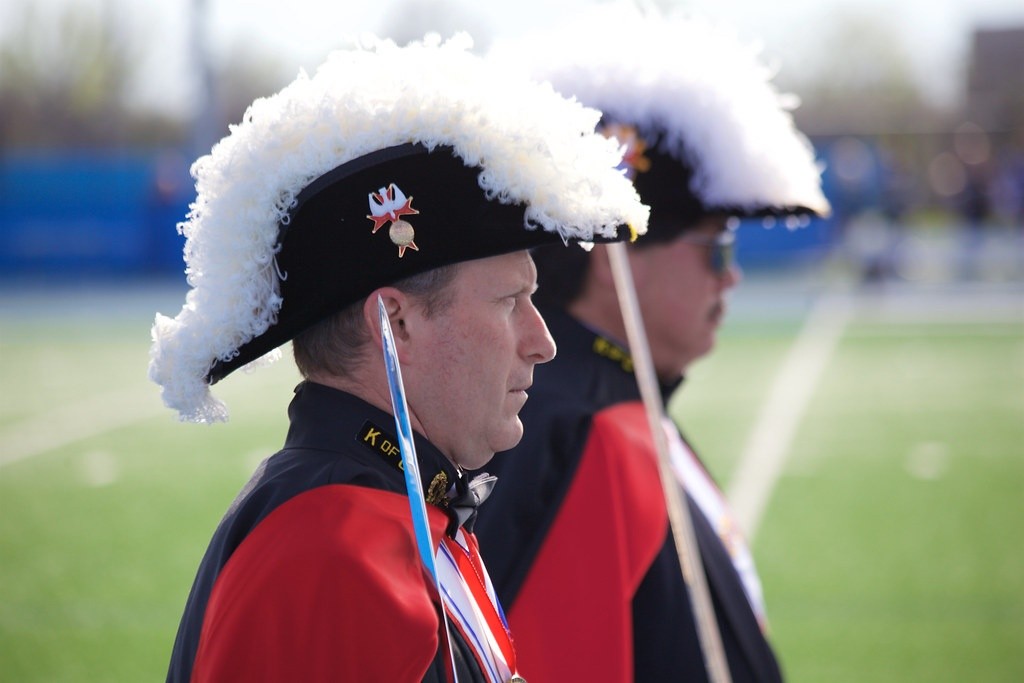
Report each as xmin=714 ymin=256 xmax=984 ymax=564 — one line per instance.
xmin=149 ymin=37 xmax=653 ymax=422
xmin=584 ymin=33 xmax=829 ymax=249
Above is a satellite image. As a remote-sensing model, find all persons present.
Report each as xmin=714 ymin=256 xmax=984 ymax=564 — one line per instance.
xmin=462 ymin=0 xmax=835 ymax=683
xmin=149 ymin=30 xmax=651 ymax=683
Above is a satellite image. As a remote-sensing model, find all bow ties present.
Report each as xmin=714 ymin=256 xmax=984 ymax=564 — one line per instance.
xmin=444 ymin=471 xmax=500 ymax=540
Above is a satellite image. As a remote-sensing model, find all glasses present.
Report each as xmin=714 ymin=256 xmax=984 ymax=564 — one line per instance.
xmin=678 ymin=228 xmax=736 ymax=273
